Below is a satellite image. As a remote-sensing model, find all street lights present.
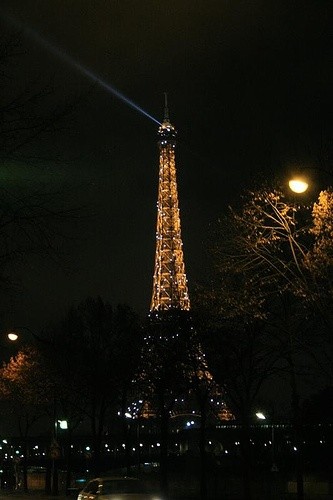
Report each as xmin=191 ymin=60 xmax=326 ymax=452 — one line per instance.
xmin=256 ymin=412 xmax=274 ymax=447
xmin=6 ymin=325 xmax=52 ymax=496
xmin=53 ymin=419 xmax=69 ymax=496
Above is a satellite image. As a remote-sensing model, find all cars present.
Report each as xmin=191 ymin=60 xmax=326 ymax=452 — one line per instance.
xmin=76 ymin=477 xmax=164 ymax=500
